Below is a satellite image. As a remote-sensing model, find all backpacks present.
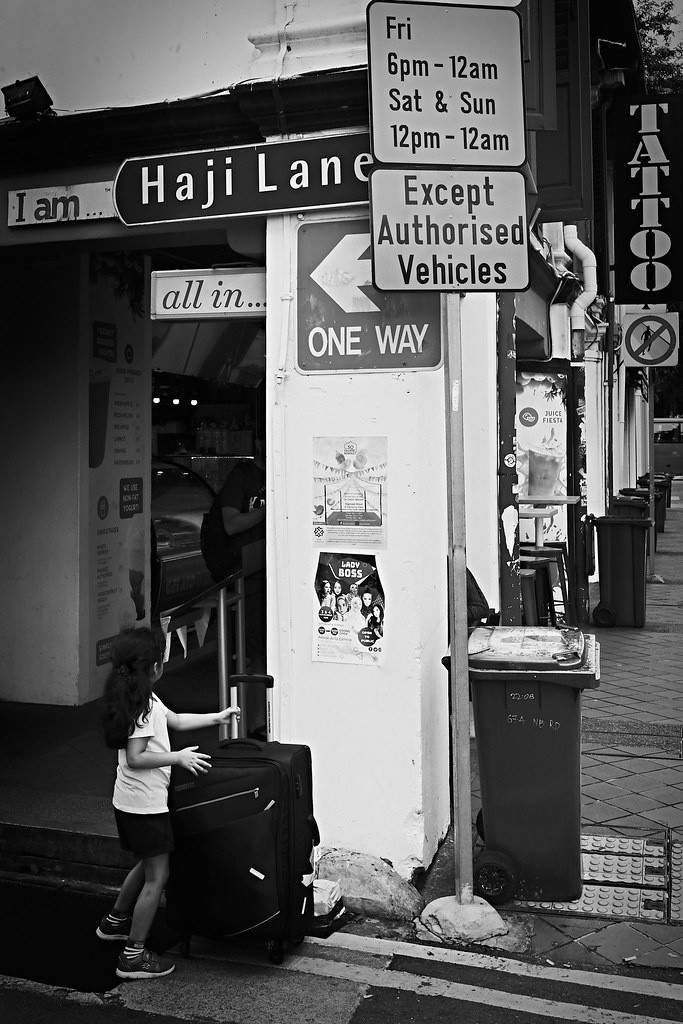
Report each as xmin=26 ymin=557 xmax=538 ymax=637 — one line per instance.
xmin=200 ymin=485 xmax=242 ymax=589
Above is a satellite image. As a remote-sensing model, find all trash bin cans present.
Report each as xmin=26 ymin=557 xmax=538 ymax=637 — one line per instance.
xmin=467 ymin=624 xmax=603 ymax=905
xmin=609 ymin=471 xmax=675 ymax=556
xmin=587 ymin=513 xmax=655 ymax=630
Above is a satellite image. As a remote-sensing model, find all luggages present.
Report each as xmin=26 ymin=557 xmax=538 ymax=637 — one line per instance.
xmin=163 ymin=674 xmax=314 ymax=966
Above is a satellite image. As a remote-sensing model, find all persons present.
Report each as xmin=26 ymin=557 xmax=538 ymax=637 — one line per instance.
xmin=92 ymin=626 xmax=242 ymax=979
xmin=195 ymin=459 xmax=268 ymax=667
xmin=311 ymin=552 xmax=388 ymax=664
xmin=439 ymin=554 xmax=491 ymax=739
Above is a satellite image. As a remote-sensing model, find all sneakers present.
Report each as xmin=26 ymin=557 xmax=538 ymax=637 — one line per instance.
xmin=95 ymin=916 xmax=132 ymax=941
xmin=116 ymin=949 xmax=175 ymax=979
